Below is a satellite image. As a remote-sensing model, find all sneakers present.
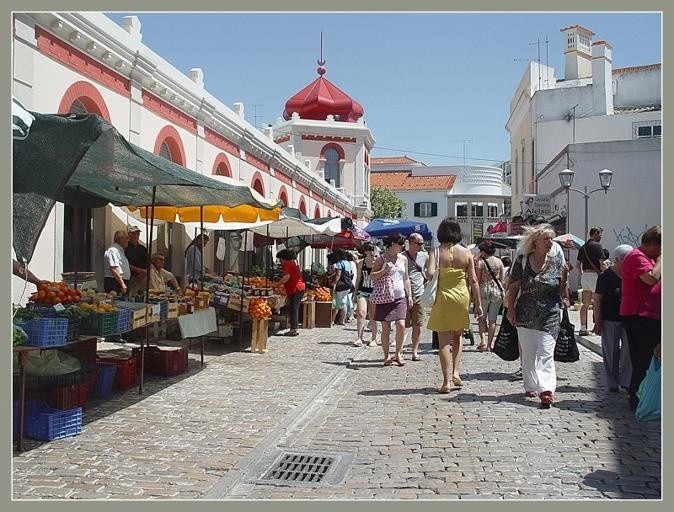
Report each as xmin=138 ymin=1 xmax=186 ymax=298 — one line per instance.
xmin=285 ymin=332 xmax=297 ymax=336
xmin=580 ymin=329 xmax=592 ymax=335
xmin=353 ymin=338 xmax=554 ymax=405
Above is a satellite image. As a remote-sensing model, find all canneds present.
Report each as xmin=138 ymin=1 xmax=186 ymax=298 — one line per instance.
xmin=187 ymin=302 xmax=194 ymax=314
xmin=179 ymin=303 xmax=187 ymax=315
xmin=243 ymin=286 xmax=273 ymax=296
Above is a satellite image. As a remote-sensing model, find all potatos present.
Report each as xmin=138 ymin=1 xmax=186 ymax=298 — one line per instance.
xmin=80 ymin=300 xmax=117 ymax=314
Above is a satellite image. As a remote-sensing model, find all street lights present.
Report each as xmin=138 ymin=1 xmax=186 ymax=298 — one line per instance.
xmin=558 ymin=168 xmax=613 ymax=241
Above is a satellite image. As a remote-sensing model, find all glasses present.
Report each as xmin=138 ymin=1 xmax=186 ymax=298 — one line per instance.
xmin=415 ymin=242 xmax=423 ymax=246
xmin=394 ymin=241 xmax=404 ymax=245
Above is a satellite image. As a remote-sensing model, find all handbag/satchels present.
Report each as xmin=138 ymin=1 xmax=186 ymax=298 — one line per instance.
xmin=332 ymin=293 xmax=343 ymax=310
xmin=554 ymin=311 xmax=579 ymax=363
xmin=335 ymin=269 xmax=353 ymax=291
xmin=368 ymin=272 xmax=395 ymax=305
xmin=420 ymin=269 xmax=439 ymax=306
xmin=493 ymin=316 xmax=519 ymax=361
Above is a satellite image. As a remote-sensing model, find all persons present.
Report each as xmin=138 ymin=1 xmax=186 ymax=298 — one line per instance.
xmin=325 ymin=218 xmax=584 ymax=408
xmin=273 ymin=249 xmax=305 ymax=336
xmin=104 ymin=230 xmax=131 ymax=299
xmin=592 ymin=245 xmax=632 ymax=394
xmin=125 ymin=225 xmax=148 ymax=291
xmin=141 ymin=252 xmax=182 ymax=293
xmin=651 ymin=343 xmax=662 ymax=362
xmin=576 ymin=227 xmax=603 ymax=336
xmin=13 ymin=260 xmax=51 ymax=291
xmin=621 ymin=226 xmax=661 ymax=405
xmin=601 ymin=249 xmax=617 ymax=273
xmin=185 ymin=234 xmax=209 ymax=284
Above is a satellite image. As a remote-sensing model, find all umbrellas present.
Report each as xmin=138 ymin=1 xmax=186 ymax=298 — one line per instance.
xmin=124 ymin=175 xmax=281 ymax=368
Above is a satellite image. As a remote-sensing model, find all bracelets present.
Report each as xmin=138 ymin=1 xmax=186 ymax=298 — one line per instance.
xmin=175 ymin=286 xmax=180 ymax=291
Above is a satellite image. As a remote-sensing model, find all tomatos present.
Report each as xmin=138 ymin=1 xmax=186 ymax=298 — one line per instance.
xmin=28 ymin=281 xmax=82 ymax=304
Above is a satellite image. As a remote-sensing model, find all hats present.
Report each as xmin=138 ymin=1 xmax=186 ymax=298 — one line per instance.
xmin=128 ymin=226 xmax=141 ymax=233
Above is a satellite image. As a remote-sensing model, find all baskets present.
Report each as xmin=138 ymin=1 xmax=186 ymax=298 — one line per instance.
xmin=14 ymin=296 xmax=185 ymax=442
